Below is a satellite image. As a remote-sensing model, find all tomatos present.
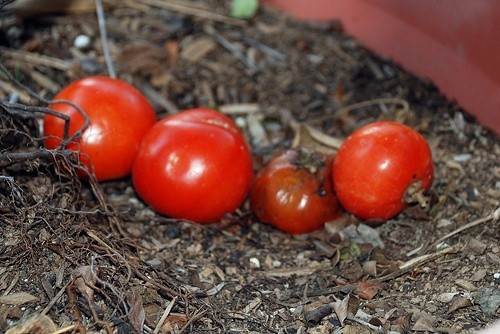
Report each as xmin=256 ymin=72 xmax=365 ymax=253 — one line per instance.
xmin=42 ymin=73 xmax=159 ymax=181
xmin=249 ymin=147 xmax=342 ymax=233
xmin=130 ymin=106 xmax=253 ymax=224
xmin=330 ymin=120 xmax=434 ymax=224
